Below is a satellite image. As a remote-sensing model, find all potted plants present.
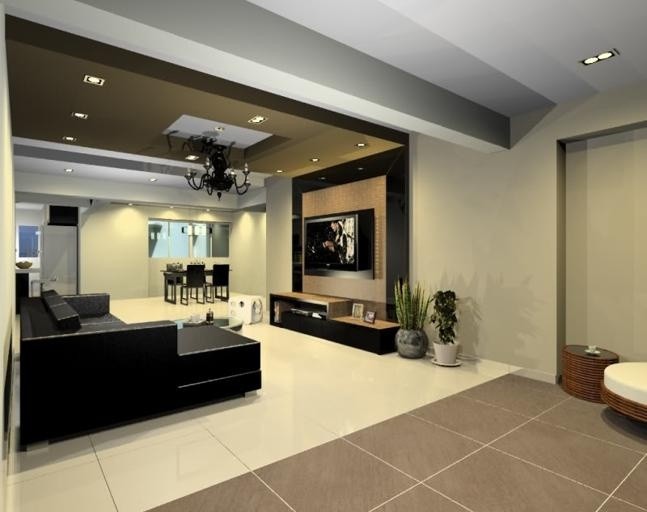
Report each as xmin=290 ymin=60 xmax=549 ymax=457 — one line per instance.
xmin=393 ymin=275 xmax=437 ymax=359
xmin=428 ymin=290 xmax=460 ymax=364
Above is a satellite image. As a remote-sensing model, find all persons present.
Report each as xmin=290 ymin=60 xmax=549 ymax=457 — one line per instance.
xmin=322 ymin=220 xmax=348 ymax=264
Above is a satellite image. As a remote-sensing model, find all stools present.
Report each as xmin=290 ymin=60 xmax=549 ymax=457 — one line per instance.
xmin=600 ymin=361 xmax=647 ymax=422
xmin=31 ymin=273 xmax=58 ymax=298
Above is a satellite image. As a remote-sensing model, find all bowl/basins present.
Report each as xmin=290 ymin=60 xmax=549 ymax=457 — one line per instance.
xmin=16 ymin=262 xmax=32 ymax=269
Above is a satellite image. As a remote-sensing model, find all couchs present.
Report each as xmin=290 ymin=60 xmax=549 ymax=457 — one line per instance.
xmin=20 ymin=290 xmax=262 ymax=452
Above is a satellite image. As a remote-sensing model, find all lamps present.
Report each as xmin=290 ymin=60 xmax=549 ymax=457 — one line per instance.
xmin=184 ymin=140 xmax=252 ymax=195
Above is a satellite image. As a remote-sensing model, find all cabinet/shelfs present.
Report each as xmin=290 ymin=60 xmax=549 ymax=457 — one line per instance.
xmin=269 ymin=291 xmax=404 ymax=355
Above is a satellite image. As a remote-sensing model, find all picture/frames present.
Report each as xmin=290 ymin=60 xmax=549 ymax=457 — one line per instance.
xmin=352 ymin=303 xmax=364 ymax=320
xmin=364 ymin=310 xmax=376 ymax=324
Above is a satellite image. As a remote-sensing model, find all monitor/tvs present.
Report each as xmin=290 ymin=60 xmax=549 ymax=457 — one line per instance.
xmin=303 ymin=208 xmax=375 ymax=281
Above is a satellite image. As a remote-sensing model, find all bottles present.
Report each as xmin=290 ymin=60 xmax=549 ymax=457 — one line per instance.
xmin=205 ymin=307 xmax=214 ymax=325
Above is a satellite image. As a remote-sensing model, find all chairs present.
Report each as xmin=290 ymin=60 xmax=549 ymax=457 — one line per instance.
xmin=165 ymin=262 xmax=230 ymax=305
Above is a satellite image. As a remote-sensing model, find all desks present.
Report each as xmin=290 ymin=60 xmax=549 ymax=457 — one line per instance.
xmin=15 ymin=267 xmax=40 ymax=314
xmin=159 ymin=269 xmax=234 ymax=302
